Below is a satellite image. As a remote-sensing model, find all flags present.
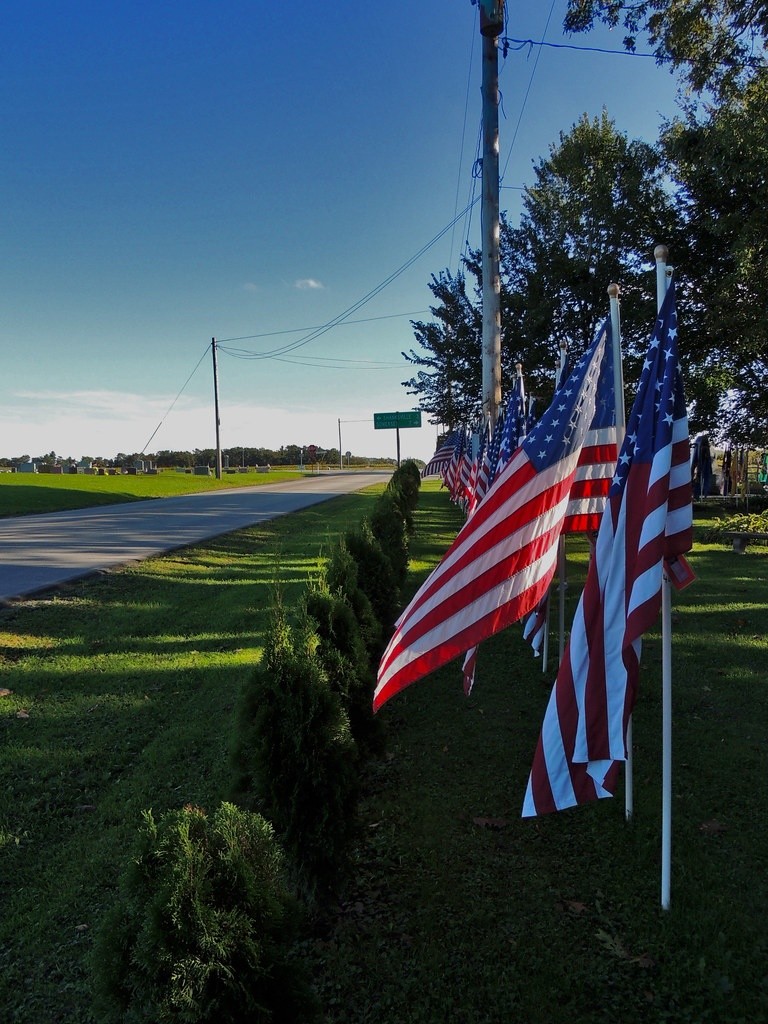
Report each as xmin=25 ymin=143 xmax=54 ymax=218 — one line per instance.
xmin=372 ymin=311 xmax=626 ymax=715
xmin=522 ymin=266 xmax=695 ymax=818
